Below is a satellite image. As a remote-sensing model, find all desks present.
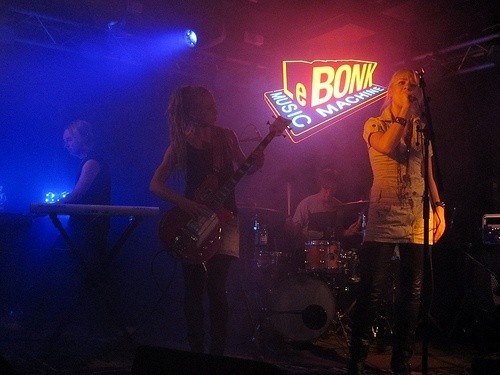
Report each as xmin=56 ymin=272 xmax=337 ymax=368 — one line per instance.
xmin=30 ymin=202 xmax=160 ymax=363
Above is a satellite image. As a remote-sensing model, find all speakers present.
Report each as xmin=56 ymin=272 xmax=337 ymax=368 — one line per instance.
xmin=130 ymin=344 xmax=287 ymax=375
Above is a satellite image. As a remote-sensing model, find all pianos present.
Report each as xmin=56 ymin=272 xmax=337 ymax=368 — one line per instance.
xmin=27 ymin=202 xmax=161 ymax=219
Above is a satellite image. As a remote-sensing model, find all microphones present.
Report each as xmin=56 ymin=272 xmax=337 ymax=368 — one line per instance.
xmin=409 ymin=95 xmax=417 ymax=103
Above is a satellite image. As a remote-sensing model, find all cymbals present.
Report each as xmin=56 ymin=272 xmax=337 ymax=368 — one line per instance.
xmin=236 ymin=206 xmax=282 ymax=216
xmin=337 ymin=200 xmax=375 ymax=206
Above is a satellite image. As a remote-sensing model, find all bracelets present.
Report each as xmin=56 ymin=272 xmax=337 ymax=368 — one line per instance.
xmin=394 ymin=116 xmax=408 ymax=127
xmin=433 ymin=201 xmax=445 ymax=208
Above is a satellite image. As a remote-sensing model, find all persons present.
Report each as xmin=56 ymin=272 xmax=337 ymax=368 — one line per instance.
xmin=290 ymin=171 xmax=359 ymax=267
xmin=149 ymin=87 xmax=265 ymax=357
xmin=346 ymin=67 xmax=447 ymax=375
xmin=56 ymin=120 xmax=113 ymax=327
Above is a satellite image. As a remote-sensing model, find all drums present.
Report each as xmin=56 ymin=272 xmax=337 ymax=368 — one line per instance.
xmin=324 ymin=248 xmax=365 ymax=299
xmin=264 ymin=270 xmax=336 ymax=344
xmin=228 ymin=247 xmax=278 ymax=303
xmin=303 ymin=239 xmax=343 ymax=272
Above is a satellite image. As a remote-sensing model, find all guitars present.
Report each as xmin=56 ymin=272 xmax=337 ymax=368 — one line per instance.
xmin=156 ymin=114 xmax=294 ymax=266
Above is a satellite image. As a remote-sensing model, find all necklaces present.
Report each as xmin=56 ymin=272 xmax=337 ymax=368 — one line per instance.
xmin=194 ymin=127 xmax=210 ymax=149
